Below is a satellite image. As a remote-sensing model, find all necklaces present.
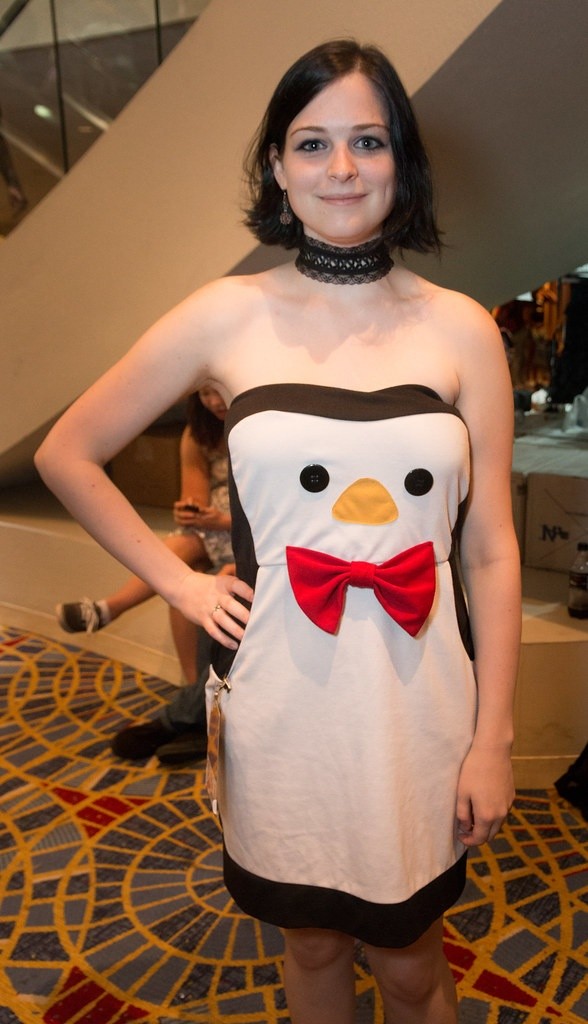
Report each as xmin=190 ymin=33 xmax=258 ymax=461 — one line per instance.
xmin=294 ymin=234 xmax=395 ymax=285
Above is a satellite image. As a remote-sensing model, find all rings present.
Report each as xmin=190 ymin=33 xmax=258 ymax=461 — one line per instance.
xmin=210 ymin=605 xmax=222 ymax=617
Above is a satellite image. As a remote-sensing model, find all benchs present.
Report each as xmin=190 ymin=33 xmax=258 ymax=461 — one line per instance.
xmin=0 ymin=489 xmax=586 ymax=788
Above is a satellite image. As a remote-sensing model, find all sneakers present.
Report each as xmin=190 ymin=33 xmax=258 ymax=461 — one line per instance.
xmin=55 ymin=601 xmax=109 ymax=632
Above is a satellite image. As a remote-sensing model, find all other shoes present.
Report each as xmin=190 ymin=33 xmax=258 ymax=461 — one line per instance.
xmin=112 ymin=726 xmax=166 ymax=761
xmin=159 ymin=737 xmax=204 ymax=761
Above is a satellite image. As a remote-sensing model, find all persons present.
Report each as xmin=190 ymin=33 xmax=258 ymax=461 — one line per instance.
xmin=33 ymin=39 xmax=522 ymax=1024
xmin=56 ymin=385 xmax=236 ymax=765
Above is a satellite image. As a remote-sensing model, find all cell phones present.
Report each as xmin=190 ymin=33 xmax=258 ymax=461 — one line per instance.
xmin=182 ymin=505 xmax=201 ymax=513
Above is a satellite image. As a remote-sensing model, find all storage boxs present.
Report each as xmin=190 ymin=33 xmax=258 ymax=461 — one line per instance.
xmin=112 ymin=416 xmax=588 ymax=571
